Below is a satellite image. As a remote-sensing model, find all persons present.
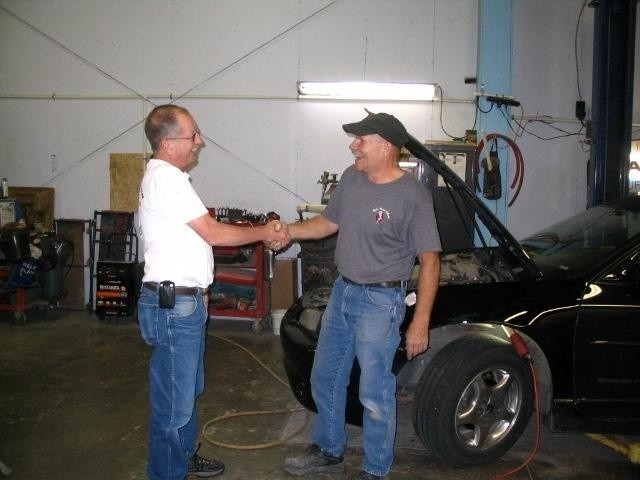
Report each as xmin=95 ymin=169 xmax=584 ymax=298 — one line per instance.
xmin=137 ymin=105 xmax=292 ymax=479
xmin=264 ymin=113 xmax=442 ymax=480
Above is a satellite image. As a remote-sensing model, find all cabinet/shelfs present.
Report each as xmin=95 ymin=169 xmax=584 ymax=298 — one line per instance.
xmin=208 ymin=207 xmax=270 ymax=333
xmin=0 ymin=270 xmax=30 ymax=327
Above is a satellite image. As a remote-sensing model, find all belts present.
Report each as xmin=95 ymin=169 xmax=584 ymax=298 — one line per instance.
xmin=340 ymin=276 xmax=416 ymax=292
xmin=143 ymin=281 xmax=215 ymax=300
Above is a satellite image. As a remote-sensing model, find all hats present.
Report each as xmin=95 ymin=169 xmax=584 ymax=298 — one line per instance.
xmin=342 ymin=113 xmax=410 ymax=149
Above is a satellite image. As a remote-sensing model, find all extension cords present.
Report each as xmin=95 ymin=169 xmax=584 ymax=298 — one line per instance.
xmin=478 ymin=93 xmax=522 ymax=107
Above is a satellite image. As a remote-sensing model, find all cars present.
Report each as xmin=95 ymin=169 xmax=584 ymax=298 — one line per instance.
xmin=280 ymin=109 xmax=639 ymax=467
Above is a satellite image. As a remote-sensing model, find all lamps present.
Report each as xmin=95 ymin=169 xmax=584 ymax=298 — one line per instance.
xmin=298 ymin=81 xmax=437 ymax=101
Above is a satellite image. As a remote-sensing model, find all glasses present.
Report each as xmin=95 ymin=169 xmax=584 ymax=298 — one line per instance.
xmin=163 ymin=128 xmax=201 ymax=141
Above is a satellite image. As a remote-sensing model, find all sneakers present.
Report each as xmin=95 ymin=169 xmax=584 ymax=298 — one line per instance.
xmin=283 ymin=443 xmax=349 ymax=477
xmin=350 ymin=470 xmax=392 ymax=480
xmin=187 ymin=453 xmax=228 ymax=476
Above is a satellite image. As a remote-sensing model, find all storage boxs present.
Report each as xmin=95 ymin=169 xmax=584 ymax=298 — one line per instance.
xmin=268 ymin=258 xmax=298 ymax=309
